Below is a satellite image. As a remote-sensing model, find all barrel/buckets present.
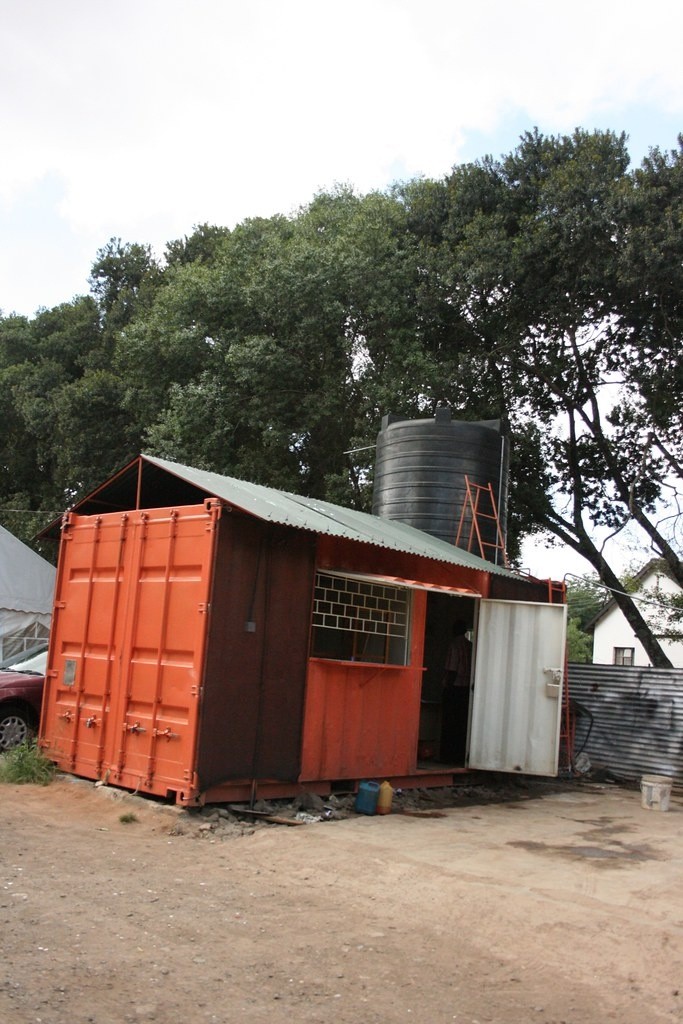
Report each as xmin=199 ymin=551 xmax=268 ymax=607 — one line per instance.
xmin=354 ymin=779 xmax=379 ymax=815
xmin=638 ymin=775 xmax=672 ymax=812
xmin=376 ymin=780 xmax=392 ymax=815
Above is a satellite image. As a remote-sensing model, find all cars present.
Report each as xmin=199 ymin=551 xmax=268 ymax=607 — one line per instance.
xmin=0 ymin=645 xmax=50 ymax=752
